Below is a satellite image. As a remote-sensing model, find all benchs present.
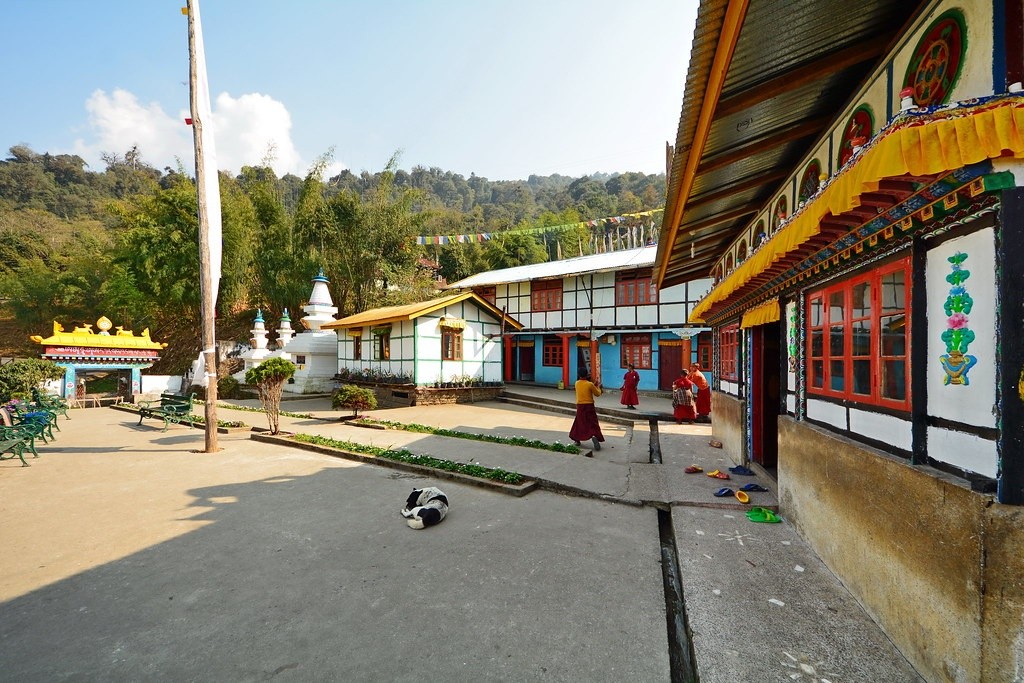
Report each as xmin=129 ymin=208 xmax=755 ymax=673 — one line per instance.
xmin=137 ymin=390 xmax=197 ymax=432
xmin=0 ymin=396 xmax=71 ymax=467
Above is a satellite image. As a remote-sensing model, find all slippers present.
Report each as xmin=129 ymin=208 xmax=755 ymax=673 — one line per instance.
xmin=746 ymin=507 xmax=775 ymax=518
xmin=735 ymin=491 xmax=749 ymax=503
xmin=728 ymin=465 xmax=755 ymax=476
xmin=748 ymin=513 xmax=782 ymax=524
xmin=684 ymin=464 xmax=705 ymax=474
xmin=740 ymin=483 xmax=769 ymax=492
xmin=706 ymin=469 xmax=730 ymax=479
xmin=713 ymin=488 xmax=735 ymax=497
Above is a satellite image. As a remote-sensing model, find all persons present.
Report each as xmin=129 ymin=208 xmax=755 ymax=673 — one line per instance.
xmin=569 ymin=367 xmax=605 ymax=451
xmin=672 ymin=363 xmax=711 ymax=425
xmin=646 ymin=237 xmax=656 ymax=246
xmin=620 ymin=363 xmax=640 ymax=410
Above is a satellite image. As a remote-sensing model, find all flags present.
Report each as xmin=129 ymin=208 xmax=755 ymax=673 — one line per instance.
xmin=416 ymin=235 xmax=477 ymax=244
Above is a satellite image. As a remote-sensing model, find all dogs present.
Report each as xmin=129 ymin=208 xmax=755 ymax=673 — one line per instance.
xmin=400 ymin=486 xmax=449 ymax=529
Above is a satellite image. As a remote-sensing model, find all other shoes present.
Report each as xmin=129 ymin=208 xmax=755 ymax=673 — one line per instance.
xmin=591 ymin=436 xmax=601 ymax=451
xmin=626 ymin=405 xmax=636 ymax=409
xmin=573 ymin=441 xmax=580 ymax=446
xmin=709 ymin=439 xmax=722 ymax=448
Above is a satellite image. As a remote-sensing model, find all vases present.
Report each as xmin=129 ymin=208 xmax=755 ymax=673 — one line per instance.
xmin=435 ymin=383 xmax=447 ymax=389
xmin=483 ymin=381 xmax=503 ymax=387
xmin=335 ymin=373 xmax=410 ymax=384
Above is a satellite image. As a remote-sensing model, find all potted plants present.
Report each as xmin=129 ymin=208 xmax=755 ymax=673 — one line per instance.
xmin=447 ymin=374 xmax=482 ymax=388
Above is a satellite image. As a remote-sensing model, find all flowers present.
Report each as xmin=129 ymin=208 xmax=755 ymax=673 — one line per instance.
xmin=6 ymin=398 xmax=30 ymax=413
xmin=339 ymin=366 xmax=413 ymax=379
